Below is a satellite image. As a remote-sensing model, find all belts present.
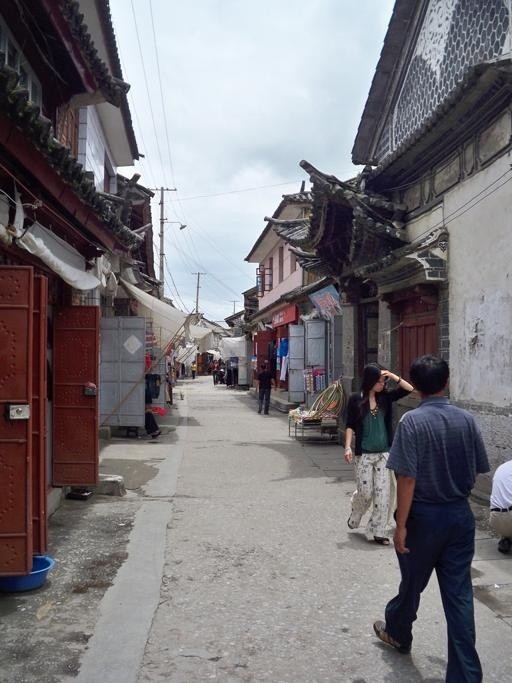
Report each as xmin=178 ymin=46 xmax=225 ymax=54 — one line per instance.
xmin=491 ymin=506 xmax=512 ymax=512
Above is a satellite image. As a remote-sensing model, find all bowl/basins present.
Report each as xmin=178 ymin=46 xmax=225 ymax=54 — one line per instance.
xmin=4 ymin=556 xmax=54 ymax=592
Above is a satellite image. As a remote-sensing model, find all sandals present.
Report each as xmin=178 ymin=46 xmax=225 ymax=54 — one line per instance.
xmin=347 ymin=514 xmax=389 ymax=545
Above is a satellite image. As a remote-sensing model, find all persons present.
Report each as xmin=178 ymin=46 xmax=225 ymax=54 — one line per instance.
xmin=373 ymin=355 xmax=490 ymax=683
xmin=191 ymin=361 xmax=197 ymax=379
xmin=490 ymin=459 xmax=512 ymax=554
xmin=343 ymin=363 xmax=415 ymax=545
xmin=212 ymin=360 xmax=220 ymax=385
xmin=256 ymin=363 xmax=276 ymax=415
xmin=146 ymin=410 xmax=161 ymax=439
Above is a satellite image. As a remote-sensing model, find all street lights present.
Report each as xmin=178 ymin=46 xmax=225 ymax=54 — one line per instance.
xmin=160 ymin=222 xmax=187 ymax=282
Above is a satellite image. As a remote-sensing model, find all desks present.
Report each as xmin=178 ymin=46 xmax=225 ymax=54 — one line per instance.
xmin=288 ymin=419 xmax=339 ymax=448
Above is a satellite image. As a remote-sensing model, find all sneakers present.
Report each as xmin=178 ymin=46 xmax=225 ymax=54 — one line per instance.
xmin=497 ymin=537 xmax=512 ymax=553
xmin=375 ymin=621 xmax=413 ymax=653
